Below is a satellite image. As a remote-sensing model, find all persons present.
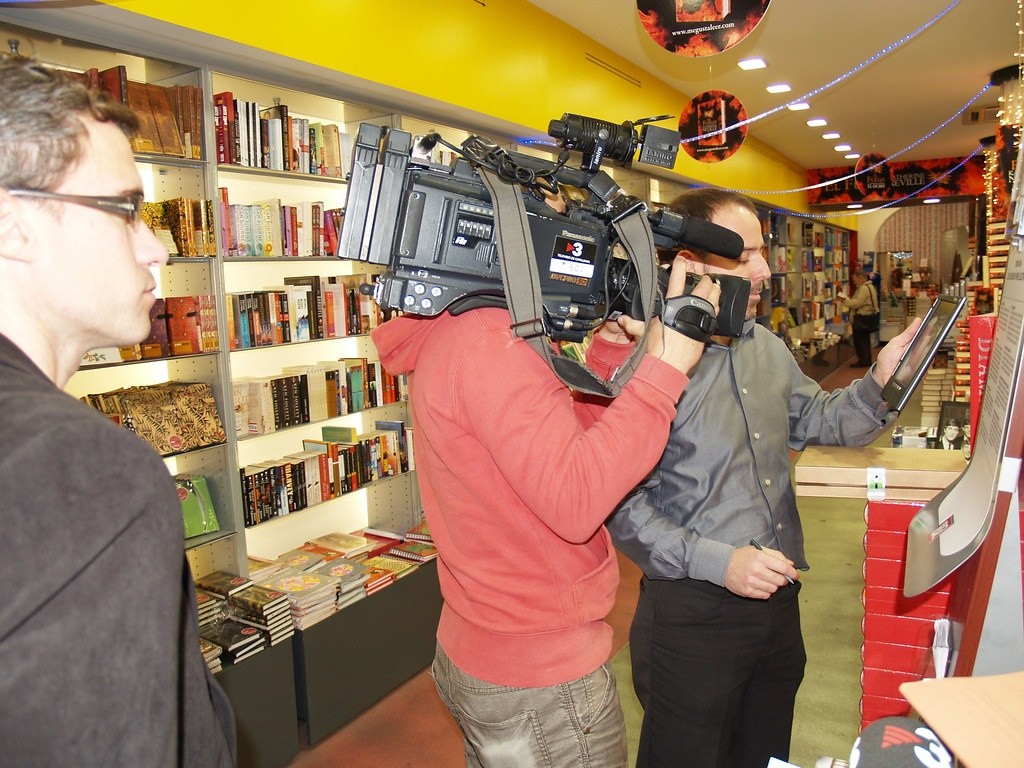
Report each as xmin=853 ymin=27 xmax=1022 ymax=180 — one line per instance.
xmin=0 ymin=56 xmax=239 ymax=768
xmin=939 ymin=419 xmax=971 ymax=459
xmin=372 ymin=180 xmax=721 ymax=768
xmin=605 ymin=189 xmax=921 ymax=768
xmin=842 ymin=271 xmax=880 ymax=368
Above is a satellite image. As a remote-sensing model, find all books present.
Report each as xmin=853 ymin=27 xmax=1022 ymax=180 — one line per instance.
xmin=755 ymin=210 xmax=853 ymax=357
xmin=909 ymin=279 xmax=1007 ymax=431
xmin=55 ymin=62 xmax=438 ymax=678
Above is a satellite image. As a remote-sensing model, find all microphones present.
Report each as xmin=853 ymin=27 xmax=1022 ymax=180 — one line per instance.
xmin=648 ymin=210 xmax=745 ymax=259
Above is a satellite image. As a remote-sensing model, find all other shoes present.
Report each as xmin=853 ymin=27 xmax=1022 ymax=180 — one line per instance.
xmin=849 ymin=360 xmax=872 ymax=367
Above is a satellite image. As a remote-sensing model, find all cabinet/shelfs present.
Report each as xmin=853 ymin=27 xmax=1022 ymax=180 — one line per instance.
xmin=0 ymin=1 xmax=858 ymax=768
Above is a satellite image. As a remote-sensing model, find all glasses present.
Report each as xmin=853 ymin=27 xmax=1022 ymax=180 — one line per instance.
xmin=9 ymin=187 xmax=145 ymax=232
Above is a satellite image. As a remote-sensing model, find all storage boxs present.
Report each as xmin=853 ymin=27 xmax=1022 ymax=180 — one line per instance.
xmin=892 ymin=425 xmax=927 ymax=448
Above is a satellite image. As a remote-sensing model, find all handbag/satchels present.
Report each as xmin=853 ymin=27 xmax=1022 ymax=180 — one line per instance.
xmin=852 ymin=312 xmax=881 ymax=333
